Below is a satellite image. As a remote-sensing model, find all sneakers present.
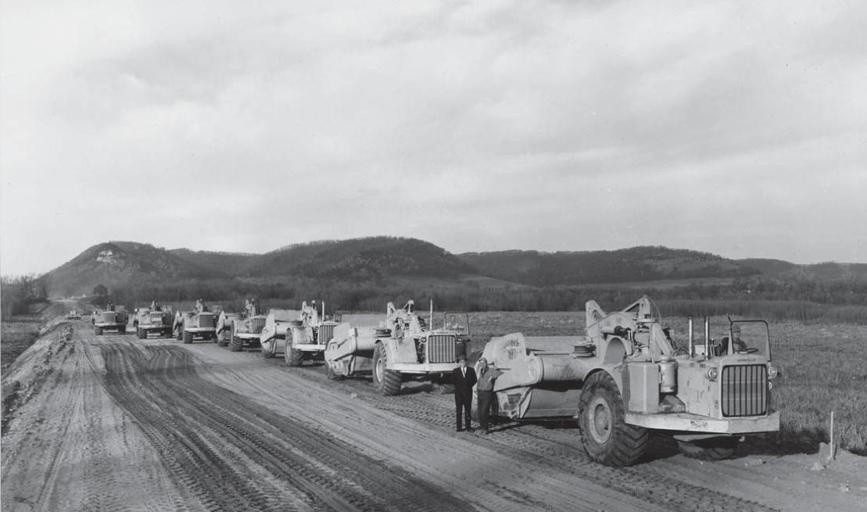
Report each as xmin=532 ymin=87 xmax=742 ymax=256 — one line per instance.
xmin=456 ymin=426 xmax=489 ymax=434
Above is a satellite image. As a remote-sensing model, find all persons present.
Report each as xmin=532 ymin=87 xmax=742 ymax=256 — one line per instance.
xmin=453 ymin=355 xmax=477 ymax=432
xmin=477 ymin=358 xmax=502 ymax=434
xmin=725 ymin=325 xmax=752 ymax=355
xmin=446 ymin=314 xmax=464 ymax=329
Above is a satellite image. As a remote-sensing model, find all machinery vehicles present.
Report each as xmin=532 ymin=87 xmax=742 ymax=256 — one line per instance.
xmin=323 ymin=301 xmax=470 ymax=395
xmin=90 ymin=298 xmax=342 ymax=372
xmin=470 ymin=294 xmax=781 ymax=467
